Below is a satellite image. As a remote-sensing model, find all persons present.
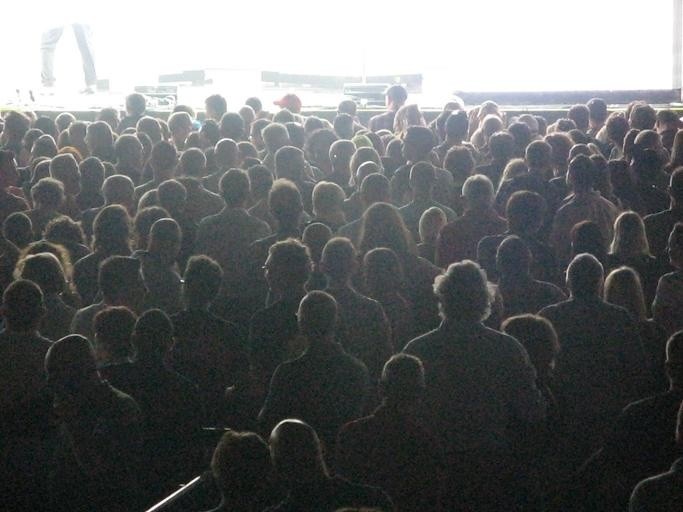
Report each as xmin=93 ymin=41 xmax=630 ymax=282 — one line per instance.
xmin=303 ymin=181 xmax=349 ymax=238
xmin=337 ymin=173 xmax=391 ymax=251
xmin=549 ymin=144 xmax=590 ymax=203
xmin=199 ymin=138 xmax=239 ymax=197
xmin=270 ymin=419 xmax=395 ymax=512
xmin=1 ymin=110 xmax=115 ymax=177
xmin=316 ymin=140 xmax=355 ymax=199
xmin=177 ymin=147 xmax=226 ymax=223
xmin=42 ymin=215 xmax=90 ymax=278
xmin=497 ymin=157 xmax=526 ymax=206
xmin=567 ymin=105 xmax=602 ymax=146
xmin=162 ymin=255 xmax=245 ymax=423
xmin=155 ymin=180 xmax=199 ymax=257
xmin=603 ymin=265 xmax=671 ymax=398
xmin=138 ymin=133 xmax=150 ymax=183
xmin=628 ymin=130 xmax=669 ymax=218
xmin=248 ymin=178 xmax=304 ymax=314
xmin=436 ymin=174 xmax=508 ymax=271
xmin=544 ymin=135 xmax=572 ymax=200
xmin=341 ymin=146 xmax=384 ymax=200
xmin=191 ymin=168 xmax=271 ymax=287
xmin=1 ymin=279 xmax=49 ymax=511
xmin=552 ymin=154 xmax=619 ymax=250
xmin=207 ymin=427 xmax=286 ymax=511
xmin=649 ymin=223 xmax=683 ymax=334
xmin=535 ymin=117 xmax=546 ymax=139
xmin=641 ymin=168 xmax=683 ymax=261
xmin=336 ymin=100 xmax=364 ymax=137
xmin=332 ymin=113 xmax=353 ymax=141
xmin=629 ymin=403 xmax=683 ymax=512
xmin=335 ymin=354 xmax=447 ymax=510
xmin=657 ymin=109 xmax=683 ymax=157
xmin=477 ymin=115 xmax=503 ymax=163
xmin=70 ymin=204 xmax=142 ymax=308
xmin=436 ymin=113 xmax=450 ymax=143
xmin=39 ymin=334 xmax=145 ymax=510
xmin=391 ymin=127 xmax=454 ymax=211
xmin=308 ymin=180 xmax=346 ymax=234
xmin=494 ymin=141 xmax=559 ymax=222
xmin=481 ymin=235 xmax=568 ymax=334
xmin=368 ymin=85 xmax=407 ymax=135
xmin=546 ymin=118 xmax=576 ymax=134
xmin=0 ymin=151 xmax=30 ymax=224
xmin=272 ymin=109 xmax=293 ymax=126
xmin=14 ymin=253 xmax=77 ymax=342
xmin=539 ymin=254 xmax=645 ymax=440
xmin=628 ymin=106 xmax=656 ymax=134
xmin=260 ymin=123 xmax=289 ymax=179
xmin=78 ymin=175 xmax=135 ymax=253
xmin=473 ymin=133 xmax=514 ymax=188
xmin=130 ymin=218 xmax=182 ymax=315
xmin=605 ymin=116 xmax=628 ymax=162
xmin=133 ymin=142 xmax=178 ymax=213
xmin=226 ymin=240 xmax=312 ymax=421
xmin=301 ymin=222 xmax=333 ymax=294
xmin=358 ymin=203 xmax=446 ymax=336
xmin=517 ymin=115 xmax=546 ymax=143
xmin=429 ymin=100 xmax=464 ymax=142
xmin=586 ymin=98 xmax=608 ymax=143
xmin=442 ymin=146 xmax=476 ymax=218
xmin=432 ymin=110 xmax=478 ymax=171
xmin=273 ymin=94 xmax=304 ymax=124
xmin=3 ymin=211 xmax=36 ymax=258
xmin=343 ymin=162 xmax=379 ymax=224
xmin=363 ymin=246 xmax=416 ymax=354
xmin=48 ymin=154 xmax=80 ymax=215
xmin=285 ymin=121 xmax=306 ymax=149
xmin=396 ymin=162 xmax=456 ymax=246
xmin=305 ymin=128 xmax=339 ymax=178
xmin=399 ymin=262 xmax=547 ymax=506
xmin=319 ymin=237 xmax=396 ymax=390
xmin=413 ymin=207 xmax=447 ymax=266
xmin=663 ymin=130 xmax=683 ymax=176
xmin=68 ymin=255 xmax=146 ymax=375
xmin=249 ymin=146 xmax=311 ymax=234
xmin=589 ymin=153 xmax=623 ymax=211
xmin=508 ymin=123 xmax=534 ymax=161
xmin=24 ymin=178 xmax=67 ymax=244
xmin=78 ymin=158 xmax=104 ymax=211
xmin=557 ymin=156 xmax=618 ymax=236
xmin=470 ymin=100 xmax=503 ymax=150
xmin=542 ymin=219 xmax=610 ymax=298
xmin=246 ymin=164 xmax=272 ymax=204
xmin=387 ymin=105 xmax=425 ymax=158
xmin=622 ymin=129 xmax=639 ymax=165
xmin=615 ymin=331 xmax=683 ymax=509
xmin=115 ymin=93 xmax=268 ymax=149
xmin=608 ymin=159 xmax=646 ymax=217
xmin=305 ymin=118 xmax=320 ymax=145
xmin=133 ymin=207 xmax=169 ymax=255
xmin=257 ymin=290 xmax=370 ymax=476
xmin=113 ymin=309 xmax=200 ymax=510
xmin=568 ymin=130 xmax=587 ymax=145
xmin=500 ymin=313 xmax=609 ymax=510
xmin=600 ymin=212 xmax=664 ymax=322
xmin=235 ymin=142 xmax=258 ymax=171
xmin=478 ymin=191 xmax=556 ymax=286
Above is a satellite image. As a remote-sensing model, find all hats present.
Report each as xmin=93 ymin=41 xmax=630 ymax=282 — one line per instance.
xmin=273 ymin=94 xmax=300 ymax=113
xmin=634 ymin=129 xmax=663 ymax=156
